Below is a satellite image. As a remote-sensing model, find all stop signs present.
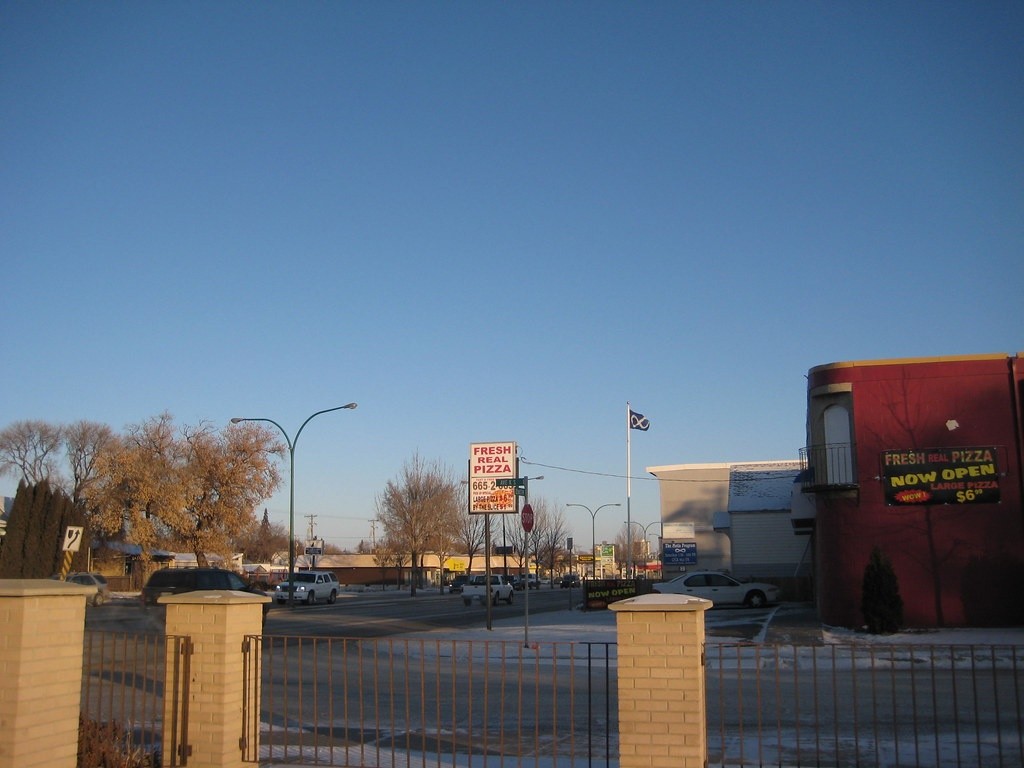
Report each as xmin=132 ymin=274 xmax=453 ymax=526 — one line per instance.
xmin=521 ymin=504 xmax=534 ymax=533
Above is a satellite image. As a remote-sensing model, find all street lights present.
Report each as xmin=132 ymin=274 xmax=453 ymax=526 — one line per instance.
xmin=566 ymin=503 xmax=622 ymax=580
xmin=460 ymin=475 xmax=545 ymax=584
xmin=624 ymin=521 xmax=661 ymax=579
xmin=230 ymin=402 xmax=358 ymax=607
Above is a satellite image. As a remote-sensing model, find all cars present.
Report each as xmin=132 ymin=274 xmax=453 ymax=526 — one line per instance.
xmin=651 ymin=571 xmax=780 ymax=608
xmin=449 ymin=576 xmax=473 ymax=593
xmin=510 ymin=573 xmax=542 ymax=590
xmin=48 ymin=572 xmax=109 ymax=607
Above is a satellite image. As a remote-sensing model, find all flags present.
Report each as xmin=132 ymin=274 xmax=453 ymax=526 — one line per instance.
xmin=629 ymin=409 xmax=649 ymax=432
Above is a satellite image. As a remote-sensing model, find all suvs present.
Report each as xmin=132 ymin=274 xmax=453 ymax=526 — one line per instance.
xmin=276 ymin=571 xmax=340 ymax=605
xmin=139 ymin=566 xmax=271 ymax=628
xmin=560 ymin=575 xmax=581 ymax=588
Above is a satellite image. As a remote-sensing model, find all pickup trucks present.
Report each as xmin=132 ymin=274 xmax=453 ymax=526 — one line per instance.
xmin=460 ymin=574 xmax=514 ymax=606
